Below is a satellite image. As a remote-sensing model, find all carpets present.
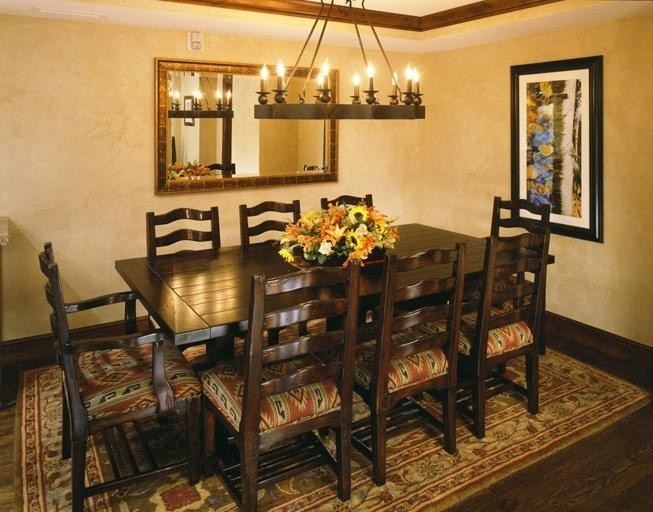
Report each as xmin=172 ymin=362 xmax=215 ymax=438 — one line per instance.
xmin=15 ymin=343 xmax=649 ymax=511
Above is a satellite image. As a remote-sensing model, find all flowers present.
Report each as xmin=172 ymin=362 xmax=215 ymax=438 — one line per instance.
xmin=166 ymin=160 xmax=217 ymax=179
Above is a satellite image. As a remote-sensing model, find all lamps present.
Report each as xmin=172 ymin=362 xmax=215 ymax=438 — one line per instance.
xmin=167 ymin=72 xmax=234 ymax=119
xmin=251 ymin=1 xmax=427 ymax=120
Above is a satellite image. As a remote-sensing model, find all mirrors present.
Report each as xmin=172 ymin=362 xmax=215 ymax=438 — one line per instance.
xmin=152 ymin=56 xmax=341 ymax=197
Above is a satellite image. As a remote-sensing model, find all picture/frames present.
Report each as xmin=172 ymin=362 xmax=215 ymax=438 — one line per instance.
xmin=183 ymin=95 xmax=194 ymax=127
xmin=508 ymin=56 xmax=605 ymax=244
